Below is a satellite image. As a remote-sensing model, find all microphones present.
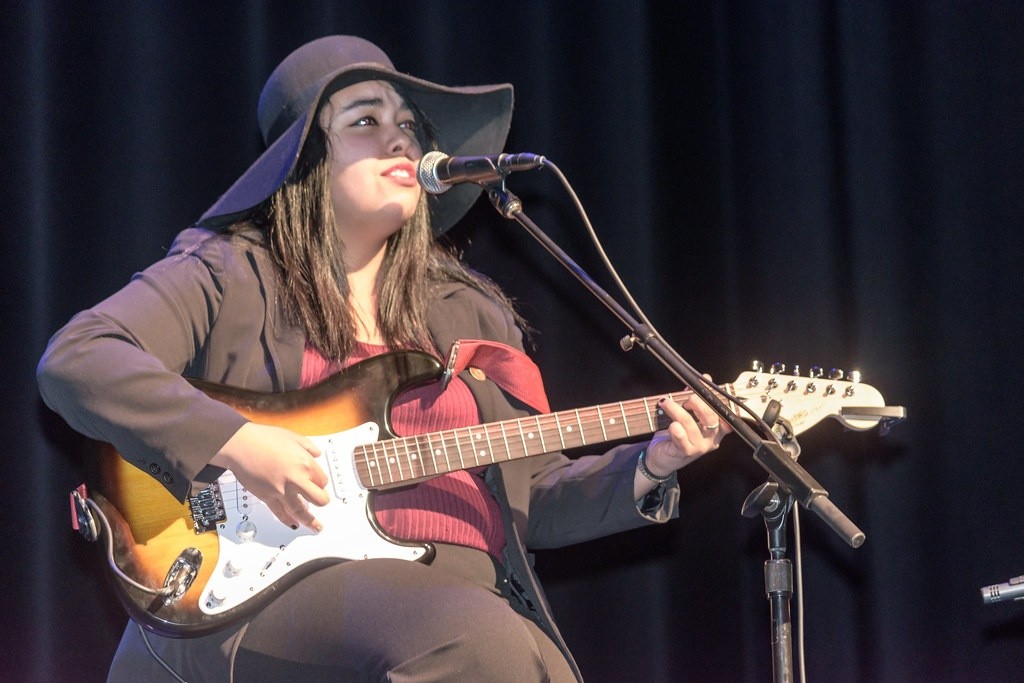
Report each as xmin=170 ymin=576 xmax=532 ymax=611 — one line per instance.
xmin=416 ymin=150 xmax=546 ymax=194
xmin=980 ymin=576 xmax=1024 ymax=605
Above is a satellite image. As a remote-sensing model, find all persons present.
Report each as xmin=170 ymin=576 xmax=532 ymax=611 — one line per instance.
xmin=35 ymin=36 xmax=734 ymax=683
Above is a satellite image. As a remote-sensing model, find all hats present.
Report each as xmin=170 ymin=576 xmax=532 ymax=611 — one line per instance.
xmin=195 ymin=35 xmax=513 ymax=242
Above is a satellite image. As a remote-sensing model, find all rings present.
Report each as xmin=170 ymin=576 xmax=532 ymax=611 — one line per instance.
xmin=704 ymin=423 xmax=720 ymax=430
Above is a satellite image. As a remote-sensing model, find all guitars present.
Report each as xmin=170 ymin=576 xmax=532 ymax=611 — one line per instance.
xmin=70 ymin=349 xmax=911 ymax=643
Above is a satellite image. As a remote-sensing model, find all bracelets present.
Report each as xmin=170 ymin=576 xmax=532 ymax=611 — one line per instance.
xmin=638 ymin=451 xmax=671 ymax=483
